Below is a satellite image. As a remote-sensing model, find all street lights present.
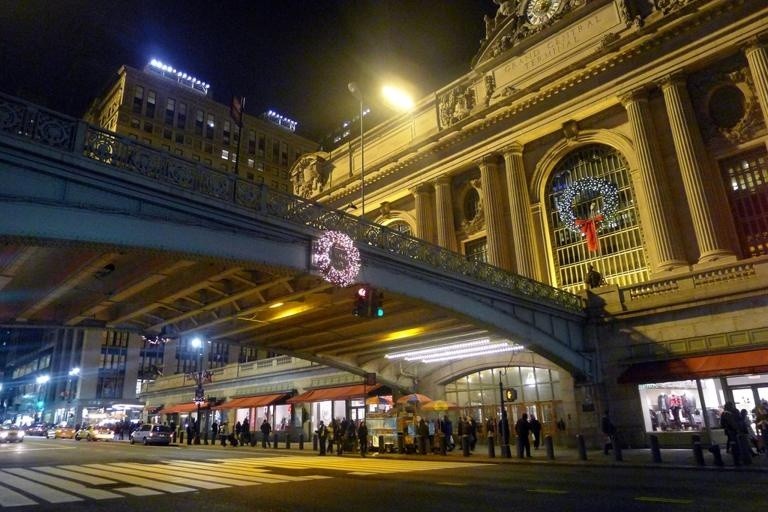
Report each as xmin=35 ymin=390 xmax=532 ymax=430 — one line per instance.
xmin=64 ymin=366 xmax=80 ymax=422
xmin=589 ymin=201 xmax=609 ymax=287
xmin=189 ymin=337 xmax=204 ymax=445
xmin=33 ymin=374 xmax=51 ymax=424
xmin=342 ymin=78 xmax=365 ymax=223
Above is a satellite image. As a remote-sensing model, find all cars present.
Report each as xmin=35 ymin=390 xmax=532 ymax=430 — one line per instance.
xmin=0 ymin=421 xmax=115 ymax=444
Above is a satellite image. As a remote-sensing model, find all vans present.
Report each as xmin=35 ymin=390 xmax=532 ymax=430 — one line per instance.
xmin=128 ymin=422 xmax=171 ymax=447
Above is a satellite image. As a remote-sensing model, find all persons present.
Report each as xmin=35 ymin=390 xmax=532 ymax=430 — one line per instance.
xmin=600 ymin=409 xmax=617 ymax=455
xmin=113 ymin=416 xmax=271 ymax=449
xmin=315 ymin=412 xmax=542 ymax=459
xmin=719 ymin=398 xmax=768 ymax=458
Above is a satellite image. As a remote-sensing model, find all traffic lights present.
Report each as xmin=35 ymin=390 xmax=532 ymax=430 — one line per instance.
xmin=504 ymin=388 xmax=517 ymax=402
xmin=351 ymin=287 xmax=370 ymax=320
xmin=369 ymin=289 xmax=384 ymax=319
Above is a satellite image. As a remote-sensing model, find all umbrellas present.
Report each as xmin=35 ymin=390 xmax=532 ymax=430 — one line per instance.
xmin=420 ymin=399 xmax=458 ymax=433
xmin=362 ymin=393 xmax=392 ymax=416
xmin=397 ymin=393 xmax=430 ymax=426
